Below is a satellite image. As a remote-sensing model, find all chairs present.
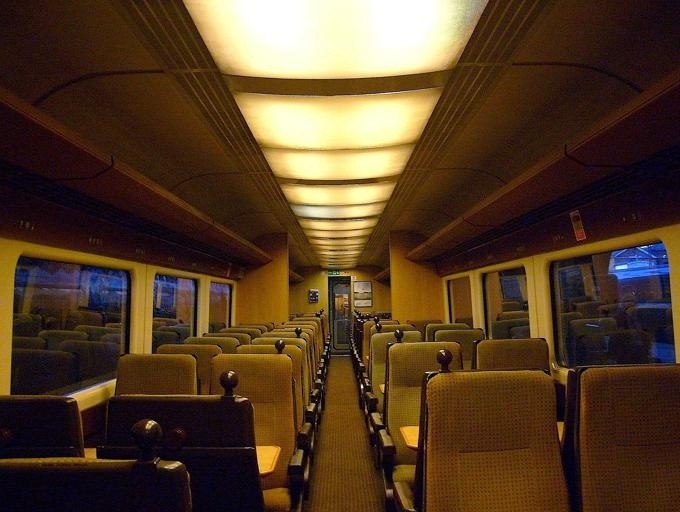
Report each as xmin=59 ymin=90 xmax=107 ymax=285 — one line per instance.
xmin=348 ymin=309 xmax=680 ymax=512
xmin=494 ymin=293 xmax=653 ymax=364
xmin=1 ymin=309 xmax=332 ymax=511
